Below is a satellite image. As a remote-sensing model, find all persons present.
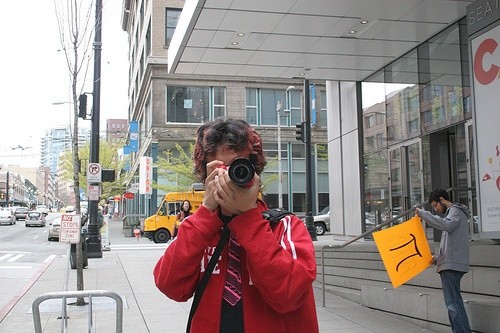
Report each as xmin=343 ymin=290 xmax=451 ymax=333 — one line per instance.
xmin=415 ymin=187 xmax=472 ymax=333
xmin=153 ymin=116 xmax=319 ymax=333
xmin=174 ymin=200 xmax=194 ymax=237
xmin=98 ymin=202 xmax=114 ymax=218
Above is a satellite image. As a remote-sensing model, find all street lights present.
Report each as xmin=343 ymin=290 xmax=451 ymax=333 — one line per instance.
xmin=276 ymin=86 xmax=295 ymax=209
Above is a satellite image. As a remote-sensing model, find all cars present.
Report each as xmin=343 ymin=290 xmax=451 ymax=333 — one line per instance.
xmin=365 ymin=211 xmax=403 ymax=227
xmin=0 ymin=207 xmax=16 ymax=225
xmin=48 ymin=219 xmax=61 ymax=240
xmin=300 ymin=206 xmax=331 ymax=236
xmin=65 ymin=201 xmax=89 ymax=228
xmin=14 ymin=207 xmax=29 ymax=221
xmin=35 ymin=205 xmax=49 ymax=216
xmin=25 ymin=211 xmax=47 ymax=227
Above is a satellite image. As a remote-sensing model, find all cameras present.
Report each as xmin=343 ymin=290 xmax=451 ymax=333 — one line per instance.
xmin=225 ymin=157 xmax=255 ymax=186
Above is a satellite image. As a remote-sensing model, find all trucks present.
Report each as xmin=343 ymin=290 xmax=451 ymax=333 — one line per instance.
xmin=142 ymin=183 xmax=267 ymax=244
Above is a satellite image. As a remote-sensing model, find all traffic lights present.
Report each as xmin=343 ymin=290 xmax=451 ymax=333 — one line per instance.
xmin=295 ymin=121 xmax=307 ymax=143
